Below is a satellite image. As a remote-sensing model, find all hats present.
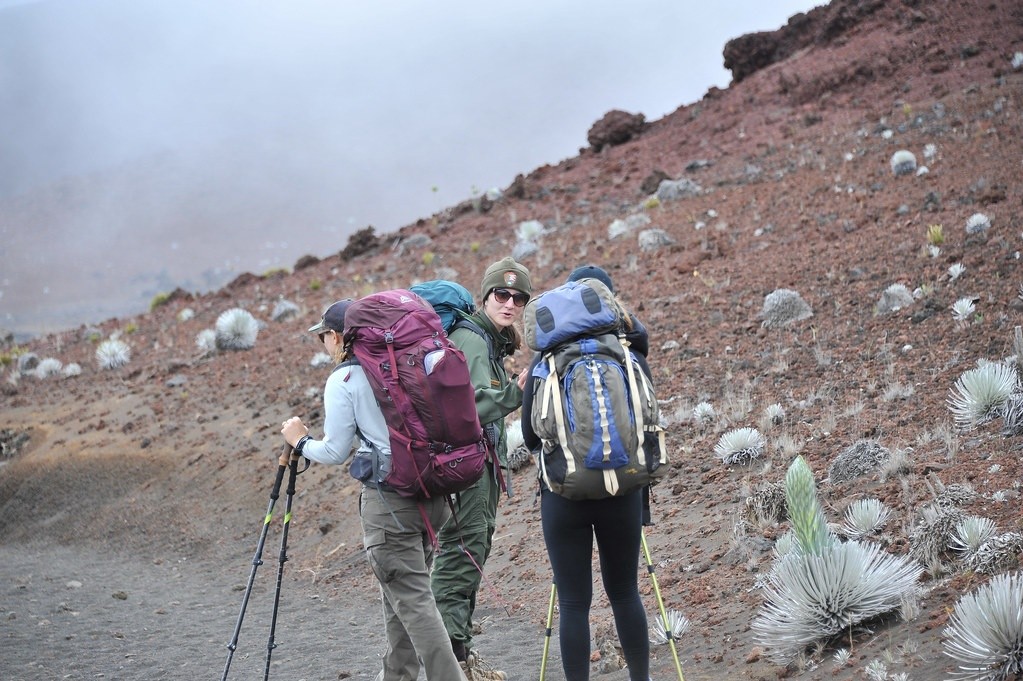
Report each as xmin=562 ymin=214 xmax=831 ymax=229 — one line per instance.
xmin=567 ymin=266 xmax=613 ymax=292
xmin=308 ymin=299 xmax=354 ymax=332
xmin=481 ymin=256 xmax=532 ymax=305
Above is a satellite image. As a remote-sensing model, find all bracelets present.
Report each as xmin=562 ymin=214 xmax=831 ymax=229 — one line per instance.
xmin=296 ymin=435 xmax=313 ymax=451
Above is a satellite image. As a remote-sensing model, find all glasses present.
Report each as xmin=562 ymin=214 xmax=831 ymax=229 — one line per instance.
xmin=317 ymin=329 xmax=330 ymax=343
xmin=493 ymin=288 xmax=530 ymax=307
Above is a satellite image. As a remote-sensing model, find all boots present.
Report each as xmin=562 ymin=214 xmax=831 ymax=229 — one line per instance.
xmin=458 ymin=649 xmax=508 ymax=681
xmin=472 ymin=621 xmax=481 ymax=635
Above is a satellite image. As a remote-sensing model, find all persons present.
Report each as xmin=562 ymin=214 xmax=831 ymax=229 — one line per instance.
xmin=521 ymin=265 xmax=654 ymax=681
xmin=429 ymin=257 xmax=531 ymax=681
xmin=281 ymin=299 xmax=469 ymax=681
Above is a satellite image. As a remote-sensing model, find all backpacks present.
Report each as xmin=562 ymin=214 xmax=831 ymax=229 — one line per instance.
xmin=523 ymin=278 xmax=670 ymax=500
xmin=333 ymin=289 xmax=489 ymax=498
xmin=409 ymin=280 xmax=493 ymax=361
